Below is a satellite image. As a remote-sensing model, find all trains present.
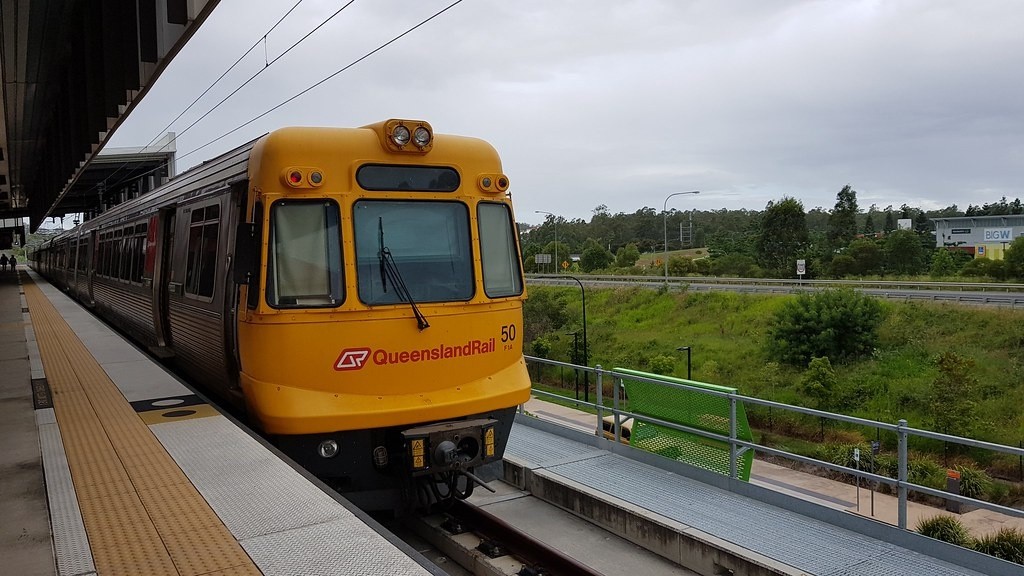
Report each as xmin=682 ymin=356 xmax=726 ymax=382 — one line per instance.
xmin=22 ymin=117 xmax=534 ymax=522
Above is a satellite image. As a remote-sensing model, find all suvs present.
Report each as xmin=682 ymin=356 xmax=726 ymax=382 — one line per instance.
xmin=595 ymin=414 xmax=683 ymax=460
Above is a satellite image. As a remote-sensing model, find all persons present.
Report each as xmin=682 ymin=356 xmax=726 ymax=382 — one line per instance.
xmin=9 ymin=255 xmax=18 ymax=271
xmin=0 ymin=253 xmax=9 ymax=271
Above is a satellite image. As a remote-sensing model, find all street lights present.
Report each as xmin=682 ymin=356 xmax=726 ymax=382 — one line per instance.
xmin=533 ymin=275 xmax=589 ymax=404
xmin=663 ymin=190 xmax=700 ymax=285
xmin=534 ymin=211 xmax=558 ymax=277
xmin=676 ymin=346 xmax=692 ymax=381
xmin=565 ymin=331 xmax=580 ymax=407
